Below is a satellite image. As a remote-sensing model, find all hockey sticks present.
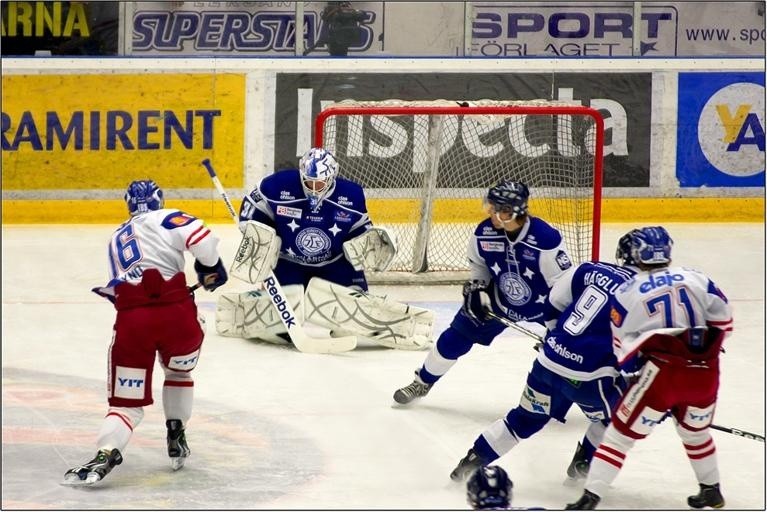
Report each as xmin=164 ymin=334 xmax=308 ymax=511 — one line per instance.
xmin=201 ymin=159 xmax=357 ymax=354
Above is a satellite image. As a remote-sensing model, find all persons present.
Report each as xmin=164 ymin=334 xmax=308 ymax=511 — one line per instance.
xmin=449 ymin=228 xmax=628 ymax=489
xmin=466 ymin=466 xmax=545 ymax=510
xmin=391 ymin=181 xmax=575 ymax=408
xmin=565 ymin=226 xmax=733 ymax=510
xmin=62 ymin=178 xmax=228 ymax=487
xmin=234 ymin=144 xmax=398 ymax=347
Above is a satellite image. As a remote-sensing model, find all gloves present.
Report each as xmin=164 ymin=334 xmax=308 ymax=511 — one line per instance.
xmin=461 ymin=282 xmax=494 ymax=327
xmin=195 ymin=259 xmax=228 ymax=292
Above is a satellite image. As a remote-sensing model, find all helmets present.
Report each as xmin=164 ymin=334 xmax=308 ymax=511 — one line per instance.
xmin=124 ymin=179 xmax=164 ymax=216
xmin=298 ymin=147 xmax=340 ymax=202
xmin=615 ymin=229 xmax=639 ymax=266
xmin=466 ymin=463 xmax=516 ymax=507
xmin=483 ymin=181 xmax=530 ymax=223
xmin=631 ymin=226 xmax=673 ymax=267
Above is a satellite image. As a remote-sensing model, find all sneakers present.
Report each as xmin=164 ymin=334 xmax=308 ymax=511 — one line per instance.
xmin=450 ymin=448 xmax=484 ymax=484
xmin=567 ymin=442 xmax=592 ymax=480
xmin=567 ymin=488 xmax=602 ymax=510
xmin=394 ymin=368 xmax=434 ymax=405
xmin=687 ymin=483 xmax=726 ymax=509
xmin=63 ymin=449 xmax=123 ymax=480
xmin=166 ymin=420 xmax=192 ymax=458
